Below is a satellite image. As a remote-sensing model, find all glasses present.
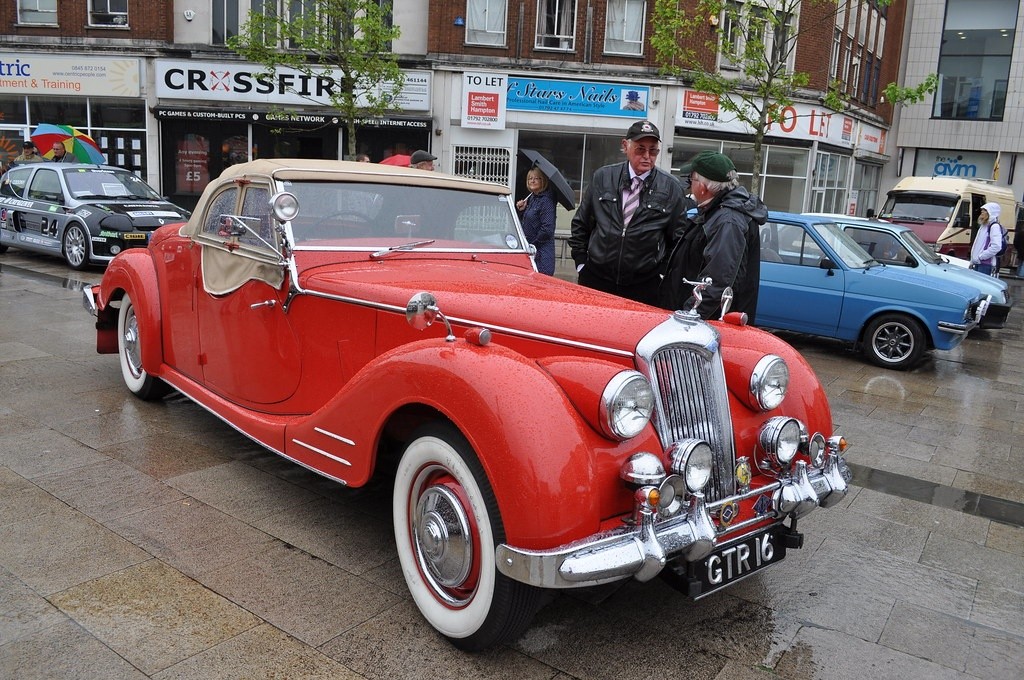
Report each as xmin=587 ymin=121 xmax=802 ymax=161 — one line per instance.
xmin=634 ymin=148 xmax=659 ymax=156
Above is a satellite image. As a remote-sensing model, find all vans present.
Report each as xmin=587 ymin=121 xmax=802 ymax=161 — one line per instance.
xmin=865 ymin=175 xmax=1017 ymax=269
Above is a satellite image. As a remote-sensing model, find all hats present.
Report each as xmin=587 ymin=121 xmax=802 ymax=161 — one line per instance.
xmin=680 ymin=150 xmax=737 ymax=182
xmin=626 ymin=121 xmax=661 ymax=142
xmin=23 ymin=141 xmax=33 ymax=147
xmin=410 ymin=150 xmax=438 ymax=164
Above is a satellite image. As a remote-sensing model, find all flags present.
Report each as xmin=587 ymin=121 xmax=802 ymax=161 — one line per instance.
xmin=993 ymin=151 xmax=1000 ymax=180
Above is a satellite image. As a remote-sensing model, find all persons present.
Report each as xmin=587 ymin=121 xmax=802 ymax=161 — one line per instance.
xmin=14 ymin=141 xmax=50 ymax=162
xmin=349 ymin=153 xmax=370 ymax=221
xmin=623 ymin=100 xmax=644 ymax=111
xmin=516 ymin=167 xmax=556 ymax=276
xmin=567 ymin=121 xmax=687 ymax=306
xmin=408 ymin=150 xmax=438 ymax=171
xmin=50 ymin=140 xmax=80 ymax=163
xmin=656 ymin=150 xmax=768 ymax=327
xmin=969 ymin=203 xmax=1002 ymax=278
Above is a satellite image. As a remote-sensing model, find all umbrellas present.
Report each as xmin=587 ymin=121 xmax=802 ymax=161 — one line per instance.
xmin=30 ymin=123 xmax=106 ymax=165
xmin=378 ymin=154 xmax=411 ymax=167
xmin=517 ymin=149 xmax=576 ymax=212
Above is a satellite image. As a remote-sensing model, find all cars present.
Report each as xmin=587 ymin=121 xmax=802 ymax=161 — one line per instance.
xmin=687 ymin=207 xmax=993 ymax=371
xmin=0 ymin=161 xmax=192 ymax=269
xmin=800 ymin=212 xmax=1014 ymax=329
xmin=81 ymin=157 xmax=856 ymax=657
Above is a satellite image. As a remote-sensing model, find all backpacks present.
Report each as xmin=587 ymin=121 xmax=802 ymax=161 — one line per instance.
xmin=989 ymin=222 xmax=1009 ymax=256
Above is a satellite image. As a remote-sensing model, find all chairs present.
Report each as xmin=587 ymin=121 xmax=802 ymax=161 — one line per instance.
xmin=760 ymin=226 xmax=786 ymax=263
